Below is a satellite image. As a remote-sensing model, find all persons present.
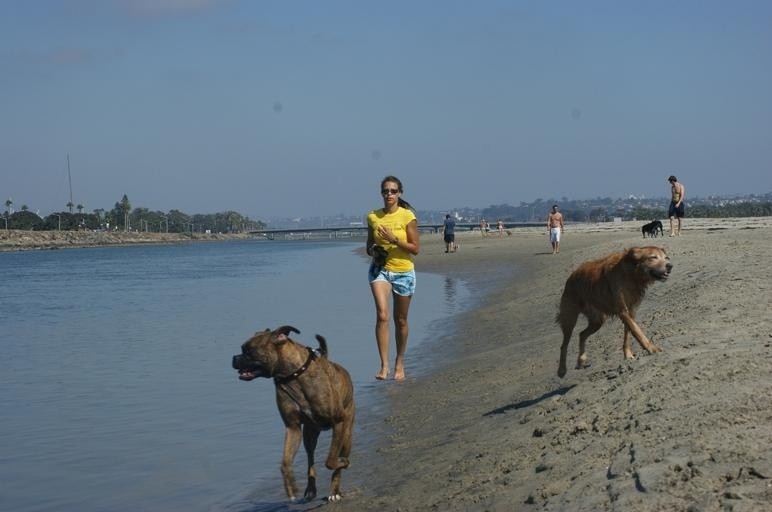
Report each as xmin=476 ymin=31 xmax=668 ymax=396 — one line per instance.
xmin=668 ymin=175 xmax=685 ymax=237
xmin=480 ymin=218 xmax=512 ymax=239
xmin=547 ymin=205 xmax=563 ymax=254
xmin=366 ymin=176 xmax=420 ymax=381
xmin=442 ymin=214 xmax=456 ymax=252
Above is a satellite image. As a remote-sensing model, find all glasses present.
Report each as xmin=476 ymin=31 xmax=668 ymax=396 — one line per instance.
xmin=383 ymin=189 xmax=398 ymax=194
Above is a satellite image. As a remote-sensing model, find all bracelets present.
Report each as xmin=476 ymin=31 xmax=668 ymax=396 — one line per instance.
xmin=392 ymin=237 xmax=400 ymax=244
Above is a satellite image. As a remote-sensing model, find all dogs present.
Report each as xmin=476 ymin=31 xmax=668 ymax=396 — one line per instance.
xmin=642 ymin=220 xmax=663 ymax=238
xmin=506 ymin=230 xmax=512 ymax=237
xmin=232 ymin=325 xmax=355 ymax=502
xmin=553 ymin=246 xmax=674 ymax=379
